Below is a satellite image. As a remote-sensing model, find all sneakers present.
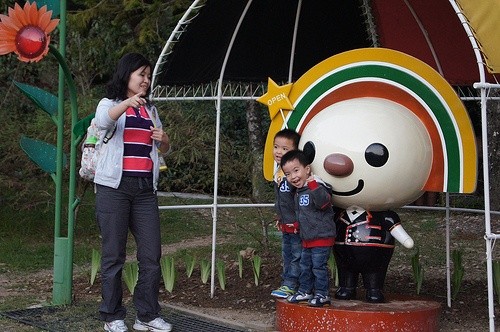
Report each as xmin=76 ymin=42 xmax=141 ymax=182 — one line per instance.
xmin=306 ymin=294 xmax=332 ymax=307
xmin=104 ymin=319 xmax=128 ymax=332
xmin=133 ymin=315 xmax=173 ymax=332
xmin=270 ymin=285 xmax=296 ymax=299
xmin=286 ymin=291 xmax=314 ymax=304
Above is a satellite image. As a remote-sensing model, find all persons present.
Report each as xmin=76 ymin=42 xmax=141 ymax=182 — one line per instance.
xmin=263 ymin=47 xmax=477 ymax=302
xmin=271 ymin=129 xmax=301 ymax=297
xmin=93 ymin=53 xmax=173 ymax=332
xmin=280 ymin=149 xmax=336 ymax=307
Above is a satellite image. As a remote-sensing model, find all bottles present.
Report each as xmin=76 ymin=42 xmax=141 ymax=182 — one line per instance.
xmin=83 ymin=136 xmax=97 ymax=160
xmin=157 ymin=149 xmax=167 ymax=172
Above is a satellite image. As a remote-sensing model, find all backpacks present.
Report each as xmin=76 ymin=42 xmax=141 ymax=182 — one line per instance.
xmin=79 ymin=117 xmax=103 ymax=180
